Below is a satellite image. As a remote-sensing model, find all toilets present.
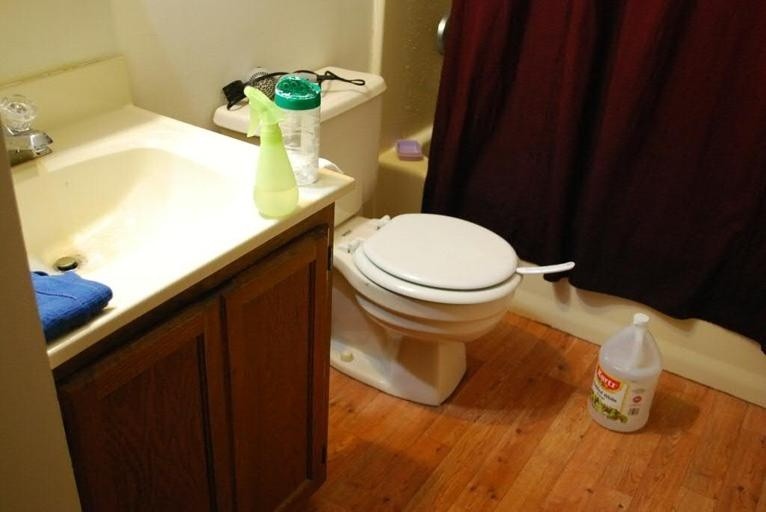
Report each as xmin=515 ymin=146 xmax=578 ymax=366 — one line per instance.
xmin=212 ymin=65 xmax=523 ymax=407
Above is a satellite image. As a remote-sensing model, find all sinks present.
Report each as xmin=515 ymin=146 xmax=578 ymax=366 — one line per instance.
xmin=11 ymin=108 xmax=354 ymax=360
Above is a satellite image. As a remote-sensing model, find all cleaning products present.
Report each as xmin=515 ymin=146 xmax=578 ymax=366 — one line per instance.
xmin=242 ymin=84 xmax=300 ymax=220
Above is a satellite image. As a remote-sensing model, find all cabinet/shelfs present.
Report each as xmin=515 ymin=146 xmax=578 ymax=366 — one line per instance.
xmin=56 ymin=224 xmax=332 ymax=512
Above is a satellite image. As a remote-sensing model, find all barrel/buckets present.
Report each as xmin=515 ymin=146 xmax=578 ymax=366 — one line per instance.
xmin=589 ymin=314 xmax=664 ymax=433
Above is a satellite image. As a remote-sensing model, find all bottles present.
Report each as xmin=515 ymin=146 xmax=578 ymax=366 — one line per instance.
xmin=271 ymin=74 xmax=323 ymax=186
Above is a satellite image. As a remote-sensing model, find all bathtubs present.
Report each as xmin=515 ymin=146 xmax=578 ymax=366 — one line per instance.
xmin=377 ymin=123 xmax=765 ymax=410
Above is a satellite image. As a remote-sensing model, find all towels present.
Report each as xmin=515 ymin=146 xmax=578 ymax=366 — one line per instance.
xmin=30 ymin=270 xmax=112 ymax=344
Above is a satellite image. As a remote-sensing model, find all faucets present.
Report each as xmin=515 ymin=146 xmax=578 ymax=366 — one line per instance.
xmin=2 ymin=97 xmax=53 ymax=162
